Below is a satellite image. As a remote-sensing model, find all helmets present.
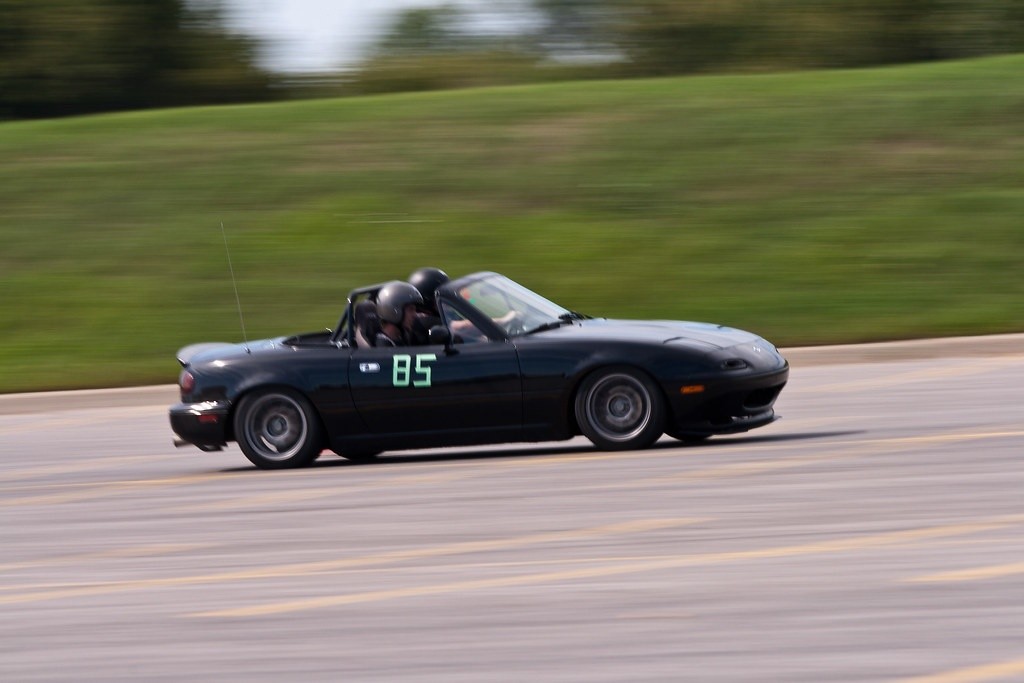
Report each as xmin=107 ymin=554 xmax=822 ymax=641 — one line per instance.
xmin=376 ymin=281 xmax=423 ymax=323
xmin=409 ymin=267 xmax=449 ymax=305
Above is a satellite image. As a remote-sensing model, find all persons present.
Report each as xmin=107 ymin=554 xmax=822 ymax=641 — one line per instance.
xmin=374 ymin=267 xmax=523 ymax=348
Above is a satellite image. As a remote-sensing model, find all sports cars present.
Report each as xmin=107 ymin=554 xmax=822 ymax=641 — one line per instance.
xmin=164 ymin=271 xmax=791 ymax=469
